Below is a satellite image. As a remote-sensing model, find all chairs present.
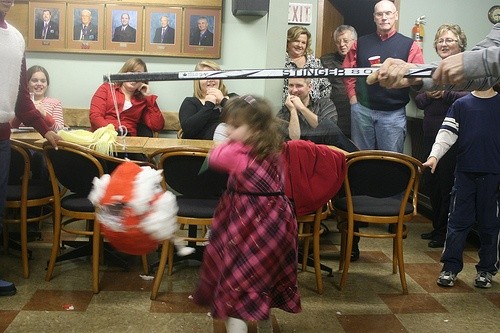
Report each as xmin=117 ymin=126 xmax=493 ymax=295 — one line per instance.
xmin=7 ymin=137 xmax=68 ymax=281
xmin=145 ymin=143 xmax=230 ymax=298
xmin=41 ymin=138 xmax=158 ymax=297
xmin=330 ymin=145 xmax=424 ymax=295
xmin=275 ymin=136 xmax=348 ymax=293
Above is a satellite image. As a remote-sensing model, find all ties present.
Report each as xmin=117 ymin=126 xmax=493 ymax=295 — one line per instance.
xmin=200 ymin=33 xmax=203 ymax=42
xmin=81 ymin=26 xmax=87 ymax=39
xmin=162 ymin=29 xmax=166 ymax=40
xmin=43 ymin=23 xmax=48 ymax=39
xmin=122 ymin=27 xmax=125 ymax=31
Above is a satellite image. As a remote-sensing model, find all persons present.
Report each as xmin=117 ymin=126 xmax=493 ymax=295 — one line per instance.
xmin=319 ymin=24 xmax=360 ymax=140
xmin=12 ymin=64 xmax=65 ymax=132
xmin=113 ymin=13 xmax=136 ymax=43
xmin=153 ymin=16 xmax=174 ymax=43
xmin=88 ymin=57 xmax=166 ymax=137
xmin=273 ymin=78 xmax=361 ymax=263
xmin=416 ymin=88 xmax=500 ymax=289
xmin=342 ymin=0 xmax=425 ymax=155
xmin=34 ymin=9 xmax=59 ymax=39
xmin=190 ymin=17 xmax=213 ymax=46
xmin=366 ymin=22 xmax=500 ymax=96
xmin=0 ymin=0 xmax=64 ymax=297
xmin=177 ymin=58 xmax=239 ymax=138
xmin=73 ymin=9 xmax=98 ymax=41
xmin=279 ymin=25 xmax=332 ymax=108
xmin=404 ymin=24 xmax=472 ymax=250
xmin=192 ymin=92 xmax=304 ymax=333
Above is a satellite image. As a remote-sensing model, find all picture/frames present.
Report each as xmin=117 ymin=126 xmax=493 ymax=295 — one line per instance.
xmin=28 ymin=0 xmax=223 ymax=59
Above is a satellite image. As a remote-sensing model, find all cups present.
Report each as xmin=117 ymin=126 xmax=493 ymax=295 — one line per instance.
xmin=368 ymin=56 xmax=380 ymax=67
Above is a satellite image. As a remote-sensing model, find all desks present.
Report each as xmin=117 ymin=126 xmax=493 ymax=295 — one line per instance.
xmin=10 ymin=128 xmax=350 ymax=277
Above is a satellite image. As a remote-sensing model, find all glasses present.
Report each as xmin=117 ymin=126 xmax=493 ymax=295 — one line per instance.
xmin=335 ymin=39 xmax=353 ymax=45
xmin=374 ymin=10 xmax=398 ymax=19
xmin=436 ymin=38 xmax=457 ymax=46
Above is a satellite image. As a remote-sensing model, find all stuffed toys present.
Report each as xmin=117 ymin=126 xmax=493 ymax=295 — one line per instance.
xmin=88 ymin=162 xmax=179 ymax=257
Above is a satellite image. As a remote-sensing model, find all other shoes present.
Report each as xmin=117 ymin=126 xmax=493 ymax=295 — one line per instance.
xmin=196 ymin=225 xmax=212 ymax=247
xmin=337 ymin=221 xmax=368 ymax=229
xmin=336 ymin=249 xmax=358 ymax=261
xmin=428 ymin=235 xmax=444 ymax=247
xmin=0 ymin=279 xmax=15 ymax=296
xmin=389 ymin=223 xmax=408 ymax=236
xmin=420 ymin=230 xmax=443 ymax=239
xmin=8 ymin=240 xmax=32 ymax=260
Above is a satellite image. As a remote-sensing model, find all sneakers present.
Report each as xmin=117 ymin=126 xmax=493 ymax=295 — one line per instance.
xmin=436 ymin=269 xmax=457 ymax=287
xmin=474 ymin=271 xmax=493 ymax=288
